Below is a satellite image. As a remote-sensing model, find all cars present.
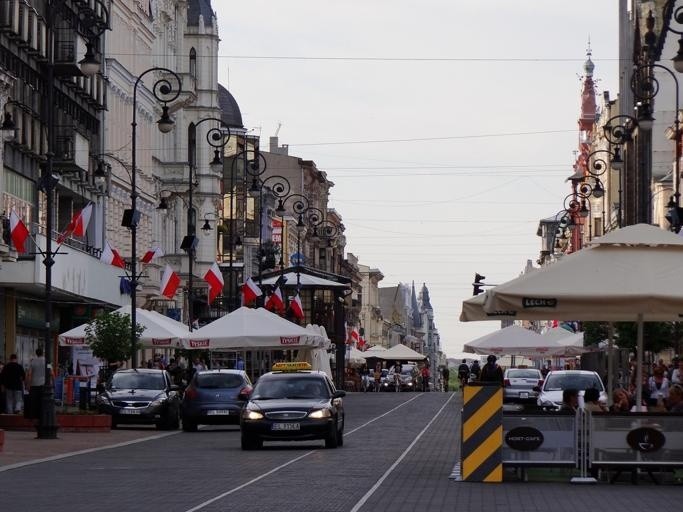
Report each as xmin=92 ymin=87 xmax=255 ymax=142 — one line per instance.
xmin=529 ymin=369 xmax=610 ymax=413
xmin=380 ymin=363 xmax=421 ymax=391
xmin=236 ymin=361 xmax=345 ymax=452
xmin=176 ymin=366 xmax=257 ymax=437
xmin=502 ymin=367 xmax=545 ymax=405
xmin=95 ymin=366 xmax=182 ymax=434
xmin=360 ymin=368 xmax=389 ymax=391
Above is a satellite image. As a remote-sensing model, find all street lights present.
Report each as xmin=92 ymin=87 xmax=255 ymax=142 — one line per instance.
xmin=222 ymin=148 xmax=342 ymax=321
xmin=153 ymin=116 xmax=232 ymax=328
xmin=547 ymin=110 xmax=640 ymax=262
xmin=622 ymin=58 xmax=683 ymax=235
xmin=89 ymin=63 xmax=183 ymax=370
xmin=0 ymin=0 xmax=115 ymax=442
xmin=659 ymin=0 xmax=682 ymax=74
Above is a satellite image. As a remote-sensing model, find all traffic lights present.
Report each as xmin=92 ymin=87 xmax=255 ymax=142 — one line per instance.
xmin=471 ymin=272 xmax=486 ymax=297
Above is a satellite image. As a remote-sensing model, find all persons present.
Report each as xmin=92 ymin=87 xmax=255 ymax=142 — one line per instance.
xmin=26 ymin=347 xmax=44 ymax=416
xmin=458 ymin=355 xmax=505 ymax=386
xmin=359 ymin=361 xmax=450 ymax=394
xmin=1 ymin=354 xmax=24 ymax=414
xmin=544 ymin=354 xmax=683 ymax=414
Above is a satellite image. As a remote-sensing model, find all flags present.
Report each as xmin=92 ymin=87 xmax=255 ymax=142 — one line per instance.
xmin=10 ymin=211 xmax=28 ymax=253
xmin=58 ymin=204 xmax=94 ymax=243
xmin=236 ymin=277 xmax=305 ymax=320
xmin=203 ymin=263 xmax=225 ymax=306
xmin=160 ymin=264 xmax=181 ymax=300
xmin=140 ymin=247 xmax=165 ymax=263
xmin=351 ymin=331 xmax=366 ymax=347
xmin=99 ymin=244 xmax=125 ymax=268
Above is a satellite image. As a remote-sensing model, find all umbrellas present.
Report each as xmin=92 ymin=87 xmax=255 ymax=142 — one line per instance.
xmin=56 ymin=306 xmax=334 ymax=382
xmin=459 ymin=274 xmax=683 ymax=401
xmin=377 ymin=344 xmax=427 ymax=360
xmin=344 ymin=346 xmax=364 ymax=367
xmin=482 ymin=223 xmax=683 ymax=472
xmin=364 ymin=345 xmax=388 ymax=357
xmin=463 ymin=325 xmax=618 ymax=367
